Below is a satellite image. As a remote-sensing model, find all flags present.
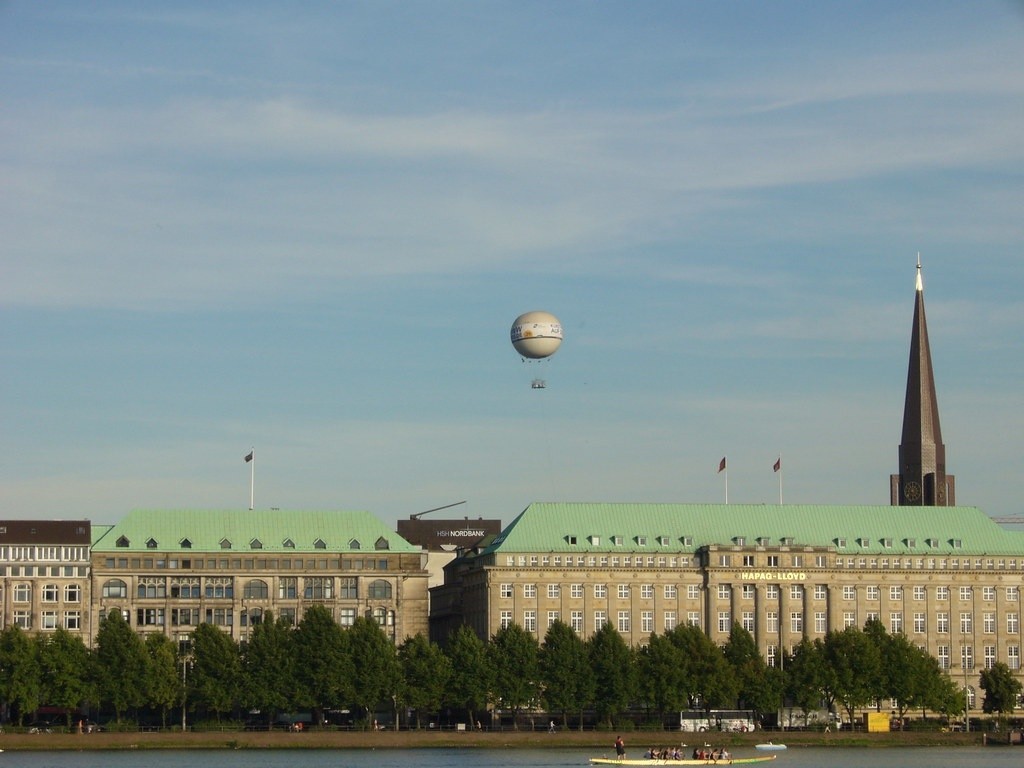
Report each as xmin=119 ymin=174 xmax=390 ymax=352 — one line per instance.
xmin=772 ymin=457 xmax=781 ymax=472
xmin=717 ymin=457 xmax=725 ymax=472
xmin=244 ymin=452 xmax=253 ymax=462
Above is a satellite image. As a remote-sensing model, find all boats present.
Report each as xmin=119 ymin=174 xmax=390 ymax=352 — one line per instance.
xmin=590 ymin=753 xmax=777 ymax=765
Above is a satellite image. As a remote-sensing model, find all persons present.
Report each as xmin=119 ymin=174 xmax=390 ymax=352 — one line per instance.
xmin=613 ymin=736 xmax=626 ymax=760
xmin=645 ymin=743 xmax=732 ymax=765
